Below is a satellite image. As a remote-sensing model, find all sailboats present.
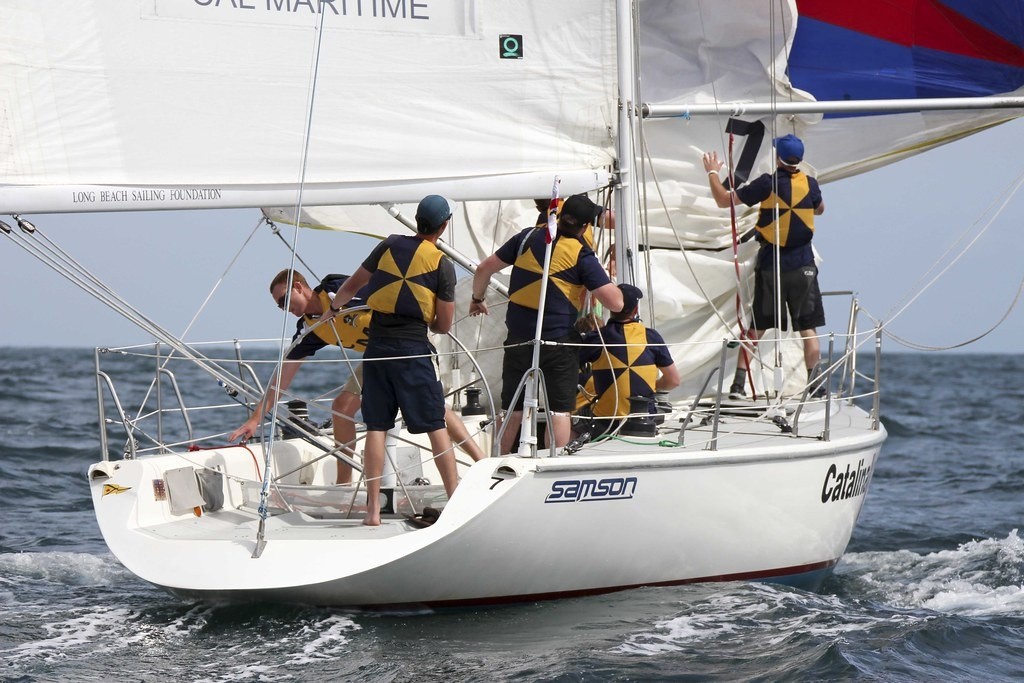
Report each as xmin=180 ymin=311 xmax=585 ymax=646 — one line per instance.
xmin=1 ymin=0 xmax=1024 ymax=608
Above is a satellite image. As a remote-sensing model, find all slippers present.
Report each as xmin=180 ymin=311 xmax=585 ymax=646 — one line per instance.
xmin=409 ymin=506 xmax=440 ymax=527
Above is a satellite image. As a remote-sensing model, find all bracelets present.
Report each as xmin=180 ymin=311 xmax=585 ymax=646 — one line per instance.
xmin=330 ymin=302 xmax=343 ymax=312
xmin=472 ymin=294 xmax=485 ymax=303
xmin=707 ymin=170 xmax=718 ymax=176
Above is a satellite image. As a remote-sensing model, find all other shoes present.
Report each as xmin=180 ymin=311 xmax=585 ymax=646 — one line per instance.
xmin=729 ymin=385 xmax=746 ymax=399
xmin=810 ymin=387 xmax=827 ymax=400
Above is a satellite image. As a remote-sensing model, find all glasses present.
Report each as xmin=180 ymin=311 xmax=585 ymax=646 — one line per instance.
xmin=278 ymin=290 xmax=289 ymax=308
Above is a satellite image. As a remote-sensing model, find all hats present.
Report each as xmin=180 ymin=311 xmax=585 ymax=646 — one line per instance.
xmin=417 ymin=195 xmax=449 ymax=233
xmin=773 ymin=134 xmax=804 ymax=166
xmin=560 ymin=195 xmax=602 ymax=228
xmin=618 ymin=284 xmax=643 ymax=313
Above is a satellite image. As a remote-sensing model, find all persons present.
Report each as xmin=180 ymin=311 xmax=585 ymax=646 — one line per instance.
xmin=228 ymin=268 xmax=485 ymax=485
xmin=704 ymin=133 xmax=826 ymax=399
xmin=470 ymin=194 xmax=679 ymax=455
xmin=320 ymin=195 xmax=459 ymax=525
